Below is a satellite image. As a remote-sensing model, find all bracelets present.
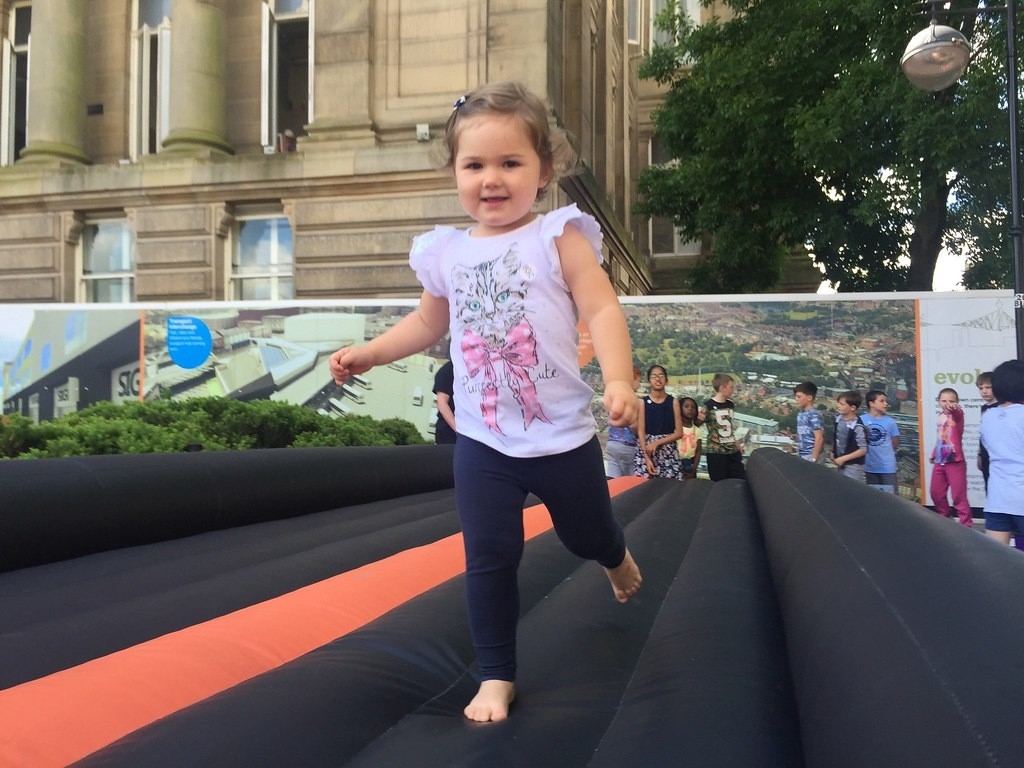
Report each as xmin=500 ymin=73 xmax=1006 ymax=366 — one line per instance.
xmin=809 ymin=458 xmax=817 ymax=462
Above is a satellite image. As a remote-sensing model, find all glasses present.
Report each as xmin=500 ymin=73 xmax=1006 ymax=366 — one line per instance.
xmin=649 ymin=374 xmax=665 ymax=380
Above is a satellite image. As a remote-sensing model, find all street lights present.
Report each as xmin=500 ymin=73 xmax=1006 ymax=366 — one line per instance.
xmin=899 ymin=1 xmax=1023 ymax=363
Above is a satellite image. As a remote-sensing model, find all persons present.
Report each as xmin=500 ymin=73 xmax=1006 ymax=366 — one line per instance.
xmin=927 ymin=388 xmax=974 ymax=527
xmin=858 ymin=391 xmax=900 ymax=496
xmin=328 ymin=85 xmax=644 ymax=725
xmin=697 ymin=374 xmax=745 ymax=482
xmin=790 ymin=381 xmax=824 ymax=463
xmin=633 ymin=364 xmax=684 ymax=482
xmin=605 ymin=364 xmax=642 ymax=481
xmin=977 ymin=360 xmax=1024 ymax=545
xmin=829 ymin=391 xmax=868 ymax=480
xmin=975 ymin=372 xmax=1001 ymax=495
xmin=674 ymin=397 xmax=703 ymax=478
xmin=433 ymin=360 xmax=458 ymax=445
